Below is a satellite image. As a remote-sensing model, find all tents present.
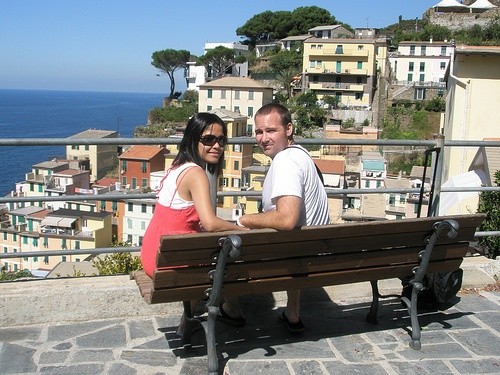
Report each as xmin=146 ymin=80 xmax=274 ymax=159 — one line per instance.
xmin=431 ymin=0 xmax=470 ymax=13
xmin=470 ymin=0 xmax=498 ymax=14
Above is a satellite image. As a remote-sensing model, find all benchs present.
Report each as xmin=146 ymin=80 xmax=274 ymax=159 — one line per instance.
xmin=126 ymin=213 xmax=488 ymax=375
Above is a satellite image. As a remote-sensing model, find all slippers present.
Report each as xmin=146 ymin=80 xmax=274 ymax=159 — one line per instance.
xmin=278 ymin=311 xmax=305 ymax=332
xmin=216 ymin=304 xmax=247 ymax=327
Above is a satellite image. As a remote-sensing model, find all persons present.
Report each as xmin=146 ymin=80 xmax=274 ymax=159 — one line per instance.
xmin=141 ymin=112 xmax=253 ymax=338
xmin=216 ymin=103 xmax=332 ymax=334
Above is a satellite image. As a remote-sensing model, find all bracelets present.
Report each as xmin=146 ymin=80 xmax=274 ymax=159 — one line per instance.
xmin=236 ymin=216 xmax=247 ymax=229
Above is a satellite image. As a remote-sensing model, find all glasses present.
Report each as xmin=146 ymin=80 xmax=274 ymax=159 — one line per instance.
xmin=199 ymin=135 xmax=227 ymax=147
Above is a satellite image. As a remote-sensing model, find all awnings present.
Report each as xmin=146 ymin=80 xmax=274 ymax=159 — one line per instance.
xmin=42 ymin=215 xmax=78 ymax=227
